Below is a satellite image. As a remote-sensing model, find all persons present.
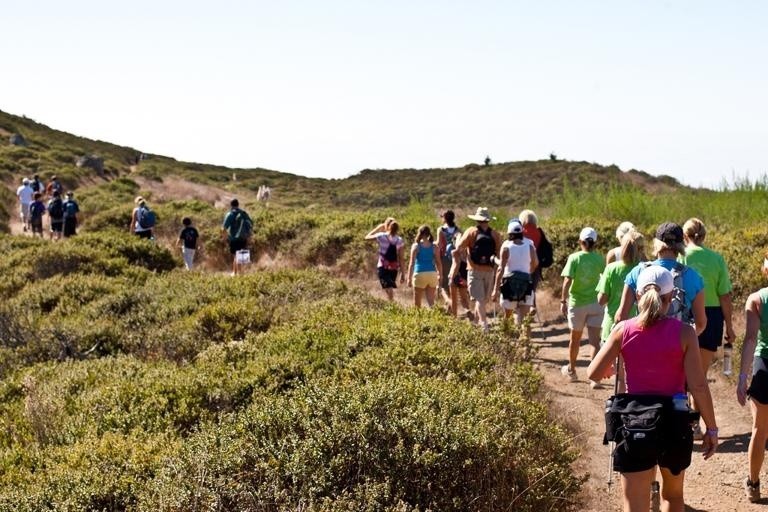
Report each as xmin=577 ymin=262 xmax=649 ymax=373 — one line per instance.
xmin=17 ymin=173 xmax=82 ymax=243
xmin=736 ymin=257 xmax=767 ymax=503
xmin=130 ymin=196 xmax=154 ymax=240
xmin=560 ymin=217 xmax=737 ymax=440
xmin=365 ymin=206 xmax=542 ymax=362
xmin=586 ymin=262 xmax=717 ymax=510
xmin=173 ymin=215 xmax=203 ymax=270
xmin=219 ymin=197 xmax=256 ymax=277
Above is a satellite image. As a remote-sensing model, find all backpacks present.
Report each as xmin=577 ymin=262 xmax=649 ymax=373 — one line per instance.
xmin=443 ymin=227 xmax=454 ymax=261
xmin=453 ymin=260 xmax=467 ymax=287
xmin=381 ymin=236 xmax=399 ymax=262
xmin=49 ymin=200 xmax=63 ymax=220
xmin=538 ymin=228 xmax=554 ymax=268
xmin=641 ymin=261 xmax=688 ymax=320
xmin=140 ymin=208 xmax=155 ymax=228
xmin=235 ymin=211 xmax=253 ymax=240
xmin=64 ymin=203 xmax=75 ymax=219
xmin=468 ymin=226 xmax=495 ymax=265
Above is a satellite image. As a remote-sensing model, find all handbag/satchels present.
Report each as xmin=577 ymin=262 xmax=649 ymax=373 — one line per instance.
xmin=606 ymin=393 xmax=669 ymax=456
xmin=501 ymin=271 xmax=529 ymax=300
xmin=236 ymin=249 xmax=252 ymax=264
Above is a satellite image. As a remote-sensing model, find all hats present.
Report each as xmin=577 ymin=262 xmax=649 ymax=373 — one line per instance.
xmin=231 ymin=198 xmax=238 ymax=206
xmin=467 ymin=207 xmax=497 ymax=222
xmin=637 ymin=265 xmax=674 ymax=296
xmin=656 ymin=222 xmax=683 ymax=243
xmin=508 ymin=209 xmax=537 ymax=234
xmin=580 ymin=227 xmax=597 ymax=242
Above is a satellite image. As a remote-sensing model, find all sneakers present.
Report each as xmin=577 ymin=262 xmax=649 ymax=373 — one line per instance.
xmin=744 ymin=476 xmax=760 ymax=502
xmin=591 ymin=381 xmax=601 ymax=389
xmin=562 ymin=365 xmax=577 ymax=380
xmin=693 ymin=425 xmax=704 ymax=440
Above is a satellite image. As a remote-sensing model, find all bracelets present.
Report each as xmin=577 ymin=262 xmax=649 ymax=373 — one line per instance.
xmin=739 ymin=373 xmax=747 ymax=379
xmin=706 ymin=426 xmax=717 ymax=439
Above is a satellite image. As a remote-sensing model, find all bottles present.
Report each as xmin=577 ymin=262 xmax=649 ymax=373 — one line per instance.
xmin=673 ymin=393 xmax=689 ymax=410
xmin=722 ymin=341 xmax=732 ymax=374
